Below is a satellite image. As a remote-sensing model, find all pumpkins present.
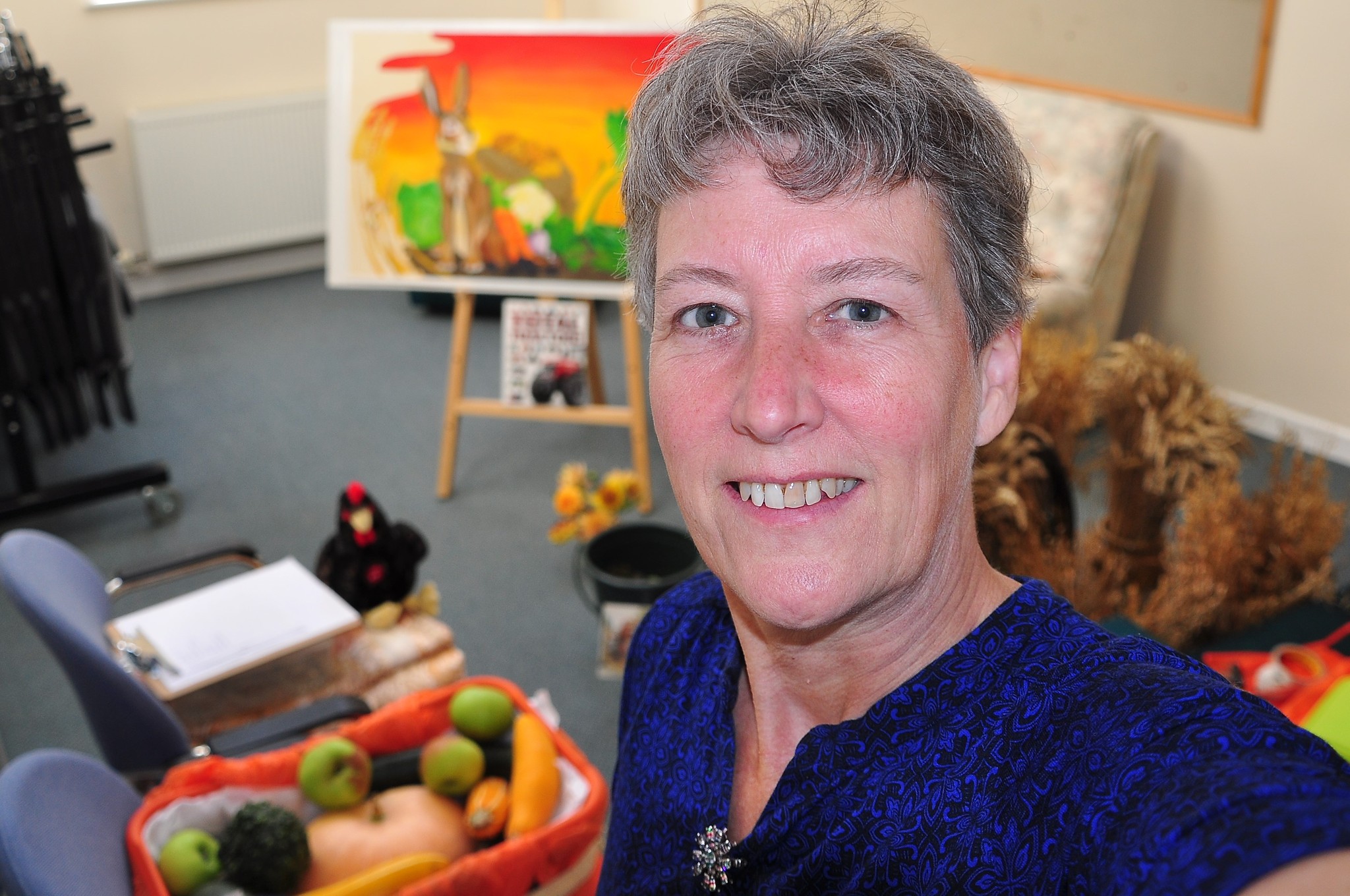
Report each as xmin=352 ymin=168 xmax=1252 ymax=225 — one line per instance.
xmin=302 ymin=786 xmax=470 ymax=890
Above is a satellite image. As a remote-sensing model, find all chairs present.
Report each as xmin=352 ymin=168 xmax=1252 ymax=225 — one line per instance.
xmin=0 ymin=730 xmax=308 ymax=896
xmin=0 ymin=529 xmax=375 ymax=790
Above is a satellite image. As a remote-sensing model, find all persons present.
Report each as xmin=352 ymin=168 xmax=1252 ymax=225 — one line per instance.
xmin=595 ymin=0 xmax=1350 ymax=896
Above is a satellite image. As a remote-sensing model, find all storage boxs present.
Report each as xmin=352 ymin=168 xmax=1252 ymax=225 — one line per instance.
xmin=170 ymin=627 xmax=361 ymax=733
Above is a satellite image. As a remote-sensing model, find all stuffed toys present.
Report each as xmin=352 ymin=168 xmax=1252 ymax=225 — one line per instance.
xmin=314 ymin=480 xmax=439 ymax=628
xmin=547 ymin=461 xmax=642 ymax=543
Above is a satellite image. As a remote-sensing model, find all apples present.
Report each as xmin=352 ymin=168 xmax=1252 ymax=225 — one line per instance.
xmin=448 ymin=686 xmax=514 ymax=737
xmin=298 ymin=739 xmax=371 ymax=809
xmin=157 ymin=829 xmax=217 ymax=894
xmin=420 ymin=736 xmax=482 ymax=794
